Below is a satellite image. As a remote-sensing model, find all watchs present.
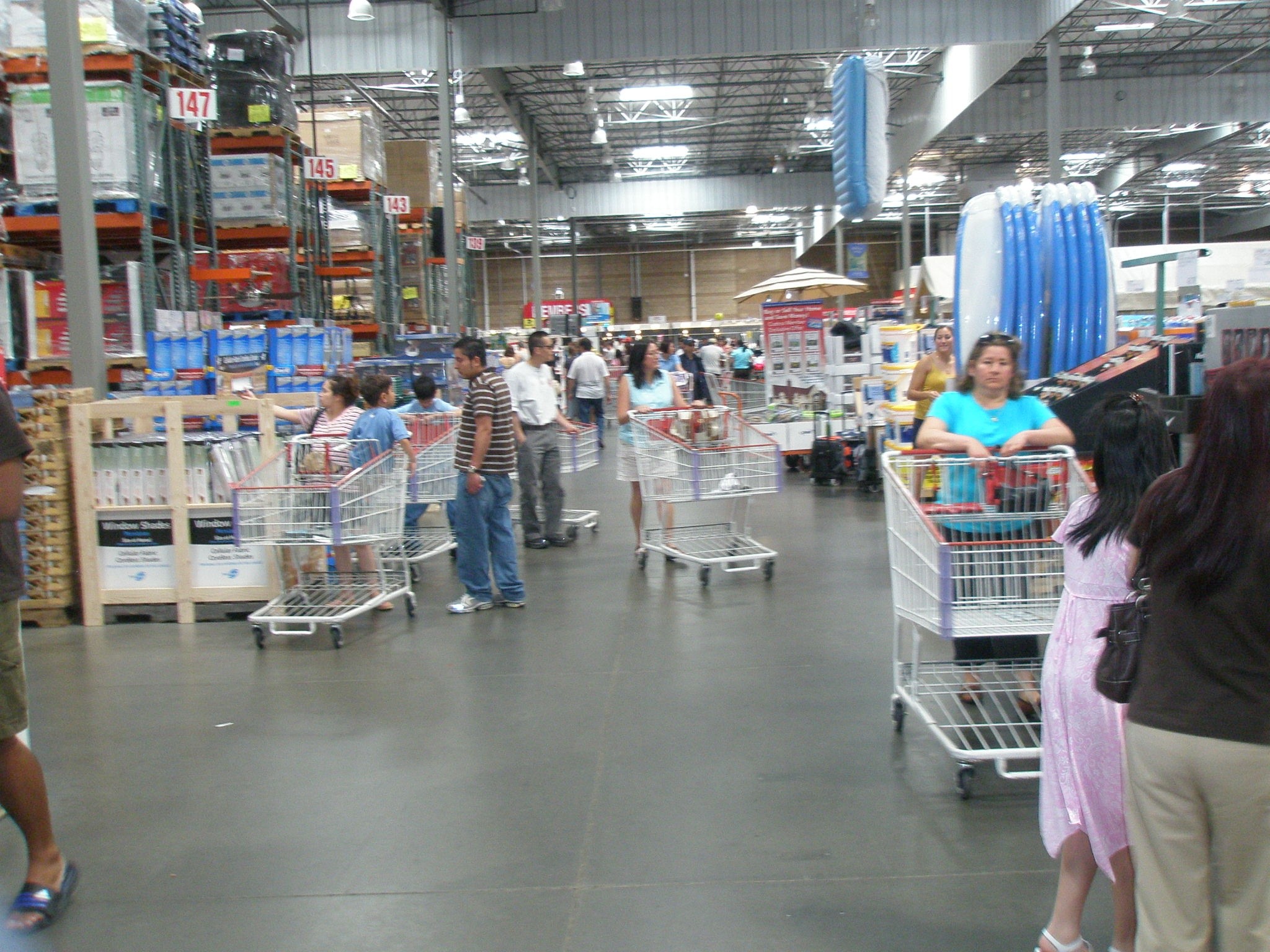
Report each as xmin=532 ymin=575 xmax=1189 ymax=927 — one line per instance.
xmin=467 ymin=464 xmax=480 ymax=473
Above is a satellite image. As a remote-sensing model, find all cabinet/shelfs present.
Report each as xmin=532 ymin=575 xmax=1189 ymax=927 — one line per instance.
xmin=212 ymin=127 xmax=309 ymax=323
xmin=322 ymin=185 xmax=388 ymax=353
xmin=402 ymin=228 xmax=430 ymax=331
xmin=457 ymin=234 xmax=477 ymax=328
xmin=311 ymin=157 xmax=336 ymax=325
xmin=6 ymin=53 xmax=181 ymax=387
xmin=426 ymin=256 xmax=463 ymax=331
xmin=388 ymin=212 xmax=400 ymax=329
xmin=0 ymin=45 xmax=465 ymax=629
xmin=64 ymin=389 xmax=328 ymax=628
xmin=169 ymin=70 xmax=223 ymax=316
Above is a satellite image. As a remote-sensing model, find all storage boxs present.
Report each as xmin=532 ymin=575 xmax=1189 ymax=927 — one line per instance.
xmin=731 ymin=315 xmax=920 ymax=455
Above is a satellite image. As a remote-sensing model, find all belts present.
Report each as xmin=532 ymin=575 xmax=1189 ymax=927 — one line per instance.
xmin=523 ymin=423 xmax=551 ymax=431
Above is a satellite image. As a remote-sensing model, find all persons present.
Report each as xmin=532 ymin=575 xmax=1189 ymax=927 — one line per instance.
xmin=916 ymin=333 xmax=1074 ymax=709
xmin=907 ymin=328 xmax=957 ymax=501
xmin=503 ymin=331 xmax=579 ymax=548
xmin=1127 ymin=361 xmax=1270 ymax=952
xmin=500 ymin=334 xmax=755 ymax=448
xmin=236 ymin=375 xmax=394 ymax=611
xmin=0 ymin=383 xmax=77 ymax=934
xmin=1034 ymin=409 xmax=1176 ymax=952
xmin=394 ymin=377 xmax=462 ymax=553
xmin=347 ymin=375 xmax=416 ymax=483
xmin=619 ymin=339 xmax=707 ymax=558
xmin=446 ymin=337 xmax=526 ymax=613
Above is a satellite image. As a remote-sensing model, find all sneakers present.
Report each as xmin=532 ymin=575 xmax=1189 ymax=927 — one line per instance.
xmin=446 ymin=594 xmax=495 ymax=613
xmin=523 ymin=537 xmax=549 ymax=548
xmin=492 ymin=593 xmax=526 ymax=608
xmin=546 ymin=536 xmax=573 ymax=547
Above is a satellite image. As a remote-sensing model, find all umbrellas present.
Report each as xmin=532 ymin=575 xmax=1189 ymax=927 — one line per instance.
xmin=734 ymin=267 xmax=872 ymax=303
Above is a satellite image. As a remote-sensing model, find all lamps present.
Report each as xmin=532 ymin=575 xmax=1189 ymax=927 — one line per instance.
xmin=562 ymin=61 xmax=586 ymax=78
xmin=346 ymin=0 xmax=378 ymax=23
xmin=625 ymin=224 xmax=637 ymax=234
xmin=771 ymin=155 xmax=786 ymax=175
xmin=784 ymin=139 xmax=800 ymax=156
xmin=607 ymin=170 xmax=623 ymax=184
xmin=590 ymin=128 xmax=608 ymax=146
xmin=598 ymin=150 xmax=614 ymax=168
xmin=517 ymin=166 xmax=531 ymax=187
xmin=581 ymin=93 xmax=599 ymax=115
xmin=535 ymin=0 xmax=566 ymax=13
xmin=453 ymin=105 xmax=471 ymax=124
xmin=1075 ymin=44 xmax=1099 ymax=79
xmin=499 ymin=157 xmax=516 ymax=172
xmin=822 ymin=66 xmax=837 ymax=90
xmin=801 ymin=96 xmax=821 ymax=126
xmin=1164 ymin=0 xmax=1190 ymax=20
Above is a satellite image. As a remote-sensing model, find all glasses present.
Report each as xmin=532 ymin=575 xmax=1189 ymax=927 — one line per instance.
xmin=538 ymin=343 xmax=555 ymax=349
xmin=646 ymin=351 xmax=663 ymax=357
xmin=979 ymin=333 xmax=1017 ymax=346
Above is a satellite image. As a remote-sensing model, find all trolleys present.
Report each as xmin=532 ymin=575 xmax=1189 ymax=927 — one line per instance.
xmin=627 ymin=403 xmax=781 ymax=586
xmin=597 ymin=365 xmax=766 ymax=440
xmin=878 ymin=444 xmax=1098 ymax=800
xmin=383 ymin=413 xmax=458 ymax=587
xmin=231 ymin=429 xmax=418 ymax=648
xmin=508 ymin=417 xmax=601 ymax=541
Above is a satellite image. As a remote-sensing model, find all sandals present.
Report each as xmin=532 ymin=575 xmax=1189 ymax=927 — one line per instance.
xmin=1033 ymin=928 xmax=1095 ymax=951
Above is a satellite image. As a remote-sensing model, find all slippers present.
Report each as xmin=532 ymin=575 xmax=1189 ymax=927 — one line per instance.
xmin=5 ymin=862 xmax=80 ymax=937
xmin=325 ymin=597 xmax=358 ymax=610
xmin=374 ymin=602 xmax=394 ymax=610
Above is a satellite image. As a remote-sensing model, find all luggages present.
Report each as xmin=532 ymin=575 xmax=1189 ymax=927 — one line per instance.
xmin=810 ymin=410 xmax=867 ymax=487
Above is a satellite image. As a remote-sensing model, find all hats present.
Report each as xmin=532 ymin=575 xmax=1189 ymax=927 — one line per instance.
xmin=683 ymin=337 xmax=696 ymax=344
xmin=708 ymin=338 xmax=717 ymax=342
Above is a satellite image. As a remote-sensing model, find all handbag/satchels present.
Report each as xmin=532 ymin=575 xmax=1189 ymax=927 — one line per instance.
xmin=995 ymin=481 xmax=1051 ymax=512
xmin=1092 ymin=592 xmax=1153 ymax=704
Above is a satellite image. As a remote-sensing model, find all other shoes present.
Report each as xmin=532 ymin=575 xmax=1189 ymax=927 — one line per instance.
xmin=1018 ymin=697 xmax=1043 ymax=715
xmin=599 ymin=439 xmax=606 ymax=448
xmin=665 ymin=547 xmax=685 ymax=560
xmin=960 ymin=686 xmax=983 ymax=704
xmin=633 ymin=545 xmax=648 ymax=557
